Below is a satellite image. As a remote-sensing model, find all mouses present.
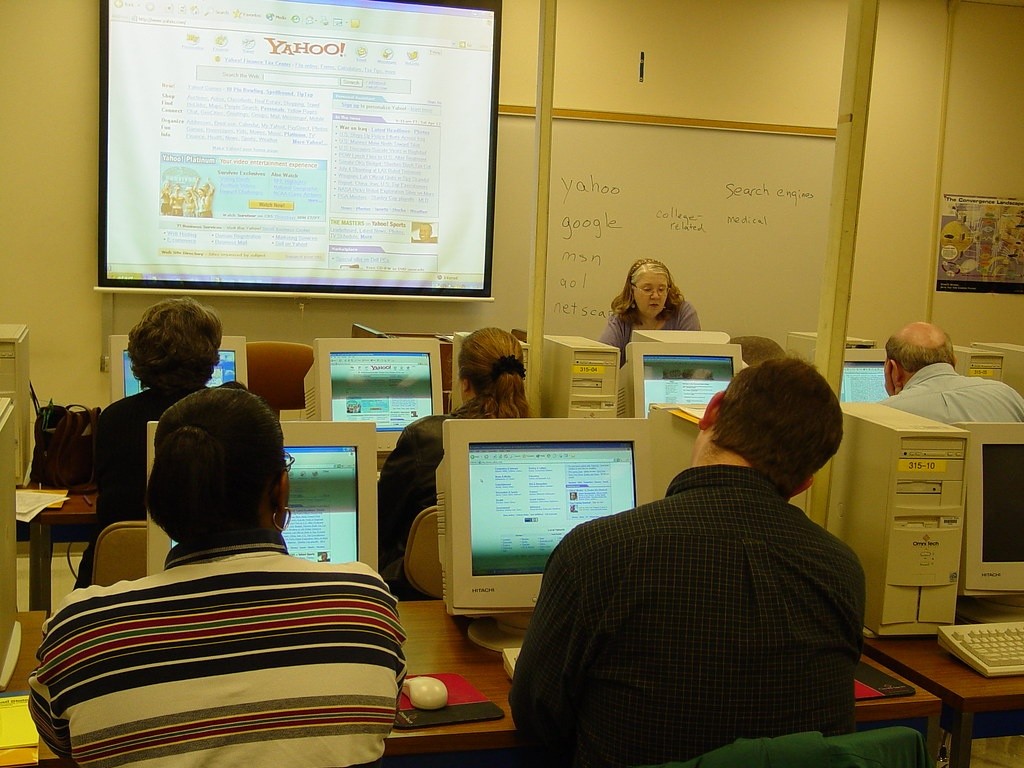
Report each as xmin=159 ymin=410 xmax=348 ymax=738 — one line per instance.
xmin=402 ymin=675 xmax=448 ymax=709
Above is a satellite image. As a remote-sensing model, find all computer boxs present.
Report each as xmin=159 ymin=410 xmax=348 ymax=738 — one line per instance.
xmin=824 ymin=400 xmax=971 ymax=635
xmin=0 ymin=320 xmax=32 ymax=688
xmin=450 ymin=332 xmax=543 ymax=420
xmin=951 ymin=344 xmax=1005 ymax=383
xmin=784 ymin=330 xmax=877 ymax=370
xmin=542 ymin=335 xmax=621 ymax=418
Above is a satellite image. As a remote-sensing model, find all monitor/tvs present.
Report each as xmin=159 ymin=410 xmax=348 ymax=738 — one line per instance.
xmin=106 ymin=333 xmax=247 ymax=409
xmin=304 ymin=338 xmax=443 ymax=455
xmin=436 ymin=417 xmax=653 ymax=653
xmin=948 ymin=420 xmax=1024 ymax=622
xmin=618 ymin=329 xmax=742 ymax=417
xmin=147 ymin=420 xmax=379 ymax=578
xmin=839 ymin=348 xmax=891 ymax=402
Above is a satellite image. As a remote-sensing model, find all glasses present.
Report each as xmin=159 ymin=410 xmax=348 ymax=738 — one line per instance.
xmin=633 ymin=285 xmax=668 ymax=296
xmin=285 ymin=452 xmax=295 ymax=472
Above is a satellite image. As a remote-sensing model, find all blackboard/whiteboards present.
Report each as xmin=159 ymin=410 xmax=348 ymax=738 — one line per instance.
xmin=101 ymin=110 xmax=532 ymax=346
xmin=542 ymin=109 xmax=843 ymax=377
xmin=553 ymin=0 xmax=851 ymax=128
xmin=497 ymin=1 xmax=543 ymax=109
xmin=847 ymin=0 xmax=954 ymax=349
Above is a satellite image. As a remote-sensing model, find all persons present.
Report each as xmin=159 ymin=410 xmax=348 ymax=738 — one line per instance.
xmin=28 ymin=388 xmax=407 ymax=768
xmin=319 ymin=553 xmax=330 ymax=563
xmin=347 ymin=402 xmax=359 ymax=413
xmin=509 ymin=358 xmax=866 ymax=768
xmin=72 ymin=296 xmax=224 ymax=591
xmin=377 ymin=328 xmax=536 ymax=603
xmin=570 ymin=492 xmax=578 ymax=512
xmin=597 ymin=258 xmax=701 ymax=369
xmin=159 ymin=176 xmax=215 ymax=218
xmin=883 ymin=321 xmax=1024 ymax=426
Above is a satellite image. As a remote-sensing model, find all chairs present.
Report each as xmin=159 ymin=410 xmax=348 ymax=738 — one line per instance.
xmin=247 ymin=341 xmax=314 ymax=409
xmin=405 ymin=504 xmax=447 ymax=598
xmin=90 ymin=522 xmax=147 ymax=586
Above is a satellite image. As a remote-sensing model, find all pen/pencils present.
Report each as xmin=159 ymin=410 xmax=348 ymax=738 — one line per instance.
xmin=639 ymin=50 xmax=645 ymax=83
xmin=82 ymin=494 xmax=93 ymax=507
xmin=39 ymin=483 xmax=41 ymax=490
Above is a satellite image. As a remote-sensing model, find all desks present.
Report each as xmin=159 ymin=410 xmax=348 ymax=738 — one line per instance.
xmin=0 ymin=491 xmax=1024 ymax=768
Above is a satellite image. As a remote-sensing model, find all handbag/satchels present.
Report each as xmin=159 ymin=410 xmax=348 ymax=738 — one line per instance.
xmin=29 ymin=404 xmax=100 ymax=496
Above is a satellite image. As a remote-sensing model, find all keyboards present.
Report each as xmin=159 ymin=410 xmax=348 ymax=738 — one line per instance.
xmin=936 ymin=620 xmax=1024 ymax=678
xmin=501 ymin=647 xmax=520 ymax=680
